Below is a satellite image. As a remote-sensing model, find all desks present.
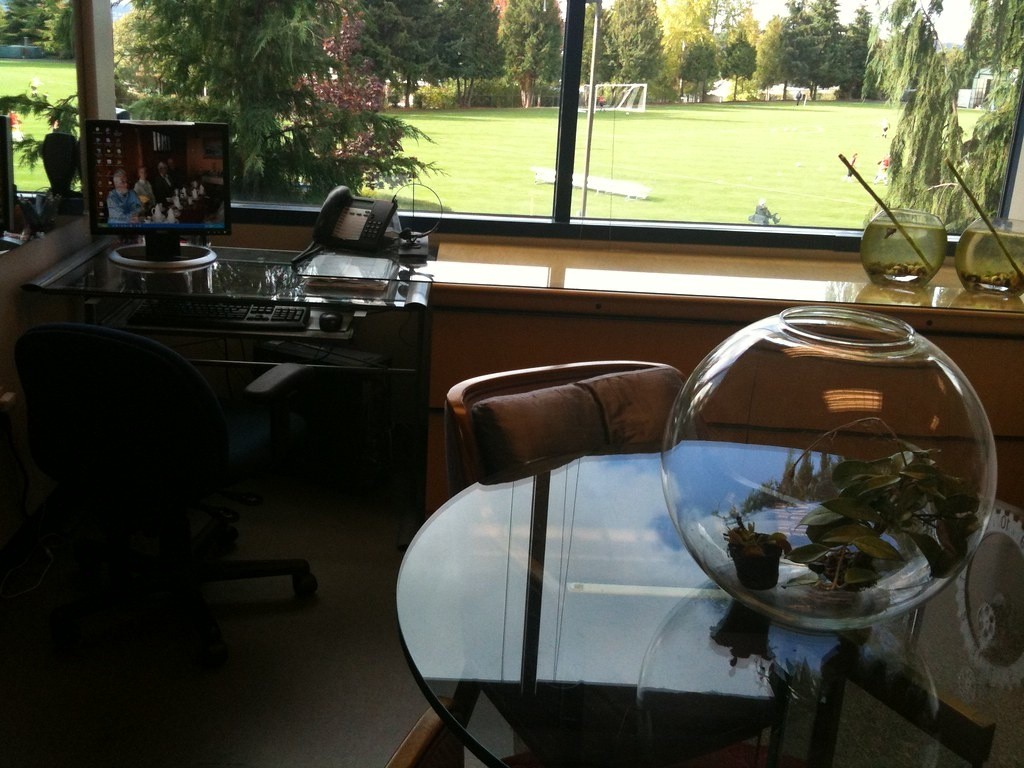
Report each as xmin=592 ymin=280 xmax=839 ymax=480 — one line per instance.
xmin=397 ymin=440 xmax=1024 ymax=768
xmin=11 ymin=238 xmax=430 ymax=523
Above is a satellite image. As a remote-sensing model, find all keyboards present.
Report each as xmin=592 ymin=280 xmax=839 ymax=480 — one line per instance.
xmin=125 ymin=296 xmax=312 ymax=333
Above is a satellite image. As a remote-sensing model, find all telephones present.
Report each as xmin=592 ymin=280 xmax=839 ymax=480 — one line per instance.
xmin=311 ymin=184 xmax=398 ymax=258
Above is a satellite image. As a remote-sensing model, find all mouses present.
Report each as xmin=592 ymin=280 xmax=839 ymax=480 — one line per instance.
xmin=320 ymin=311 xmax=343 ymax=332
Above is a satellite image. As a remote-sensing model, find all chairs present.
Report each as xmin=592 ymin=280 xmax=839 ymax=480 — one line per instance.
xmin=14 ymin=326 xmax=319 ymax=669
xmin=385 ymin=357 xmax=749 ymax=768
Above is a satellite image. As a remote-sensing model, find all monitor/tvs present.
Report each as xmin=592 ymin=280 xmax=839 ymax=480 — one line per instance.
xmin=82 ymin=119 xmax=232 ymax=270
xmin=0 ymin=115 xmax=15 ymax=233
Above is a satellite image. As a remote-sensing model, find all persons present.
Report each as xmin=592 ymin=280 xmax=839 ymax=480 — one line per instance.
xmin=134 ymin=167 xmax=158 ymax=214
xmin=107 ymin=169 xmax=143 ymax=223
xmin=151 ymin=161 xmax=175 ymax=203
xmin=599 ymin=94 xmax=606 ymax=112
